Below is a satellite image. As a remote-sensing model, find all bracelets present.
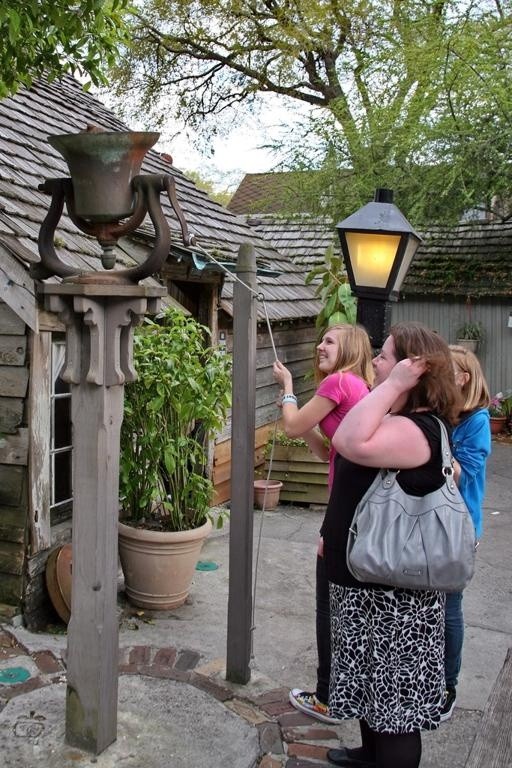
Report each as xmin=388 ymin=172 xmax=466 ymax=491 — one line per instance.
xmin=281 ymin=394 xmax=297 ymax=406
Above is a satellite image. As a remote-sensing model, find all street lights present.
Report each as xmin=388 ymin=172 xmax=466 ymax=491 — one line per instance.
xmin=335 ymin=188 xmax=423 ymax=354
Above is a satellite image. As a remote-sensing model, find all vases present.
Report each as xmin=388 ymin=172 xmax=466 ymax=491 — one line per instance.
xmin=490 ymin=415 xmax=507 ymax=434
xmin=253 ymin=480 xmax=284 ymax=510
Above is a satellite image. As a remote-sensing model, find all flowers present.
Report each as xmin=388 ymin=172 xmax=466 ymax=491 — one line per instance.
xmin=489 ymin=392 xmax=505 ymax=417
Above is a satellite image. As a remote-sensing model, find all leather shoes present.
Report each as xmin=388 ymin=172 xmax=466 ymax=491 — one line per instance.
xmin=326 ymin=745 xmax=377 ymax=768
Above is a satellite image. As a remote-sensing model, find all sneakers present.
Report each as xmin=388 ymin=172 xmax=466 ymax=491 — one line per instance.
xmin=434 ymin=687 xmax=457 ymax=723
xmin=287 ymin=680 xmax=345 ymax=726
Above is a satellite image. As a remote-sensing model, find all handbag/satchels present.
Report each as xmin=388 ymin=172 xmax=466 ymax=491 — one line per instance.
xmin=342 ymin=411 xmax=479 ymax=594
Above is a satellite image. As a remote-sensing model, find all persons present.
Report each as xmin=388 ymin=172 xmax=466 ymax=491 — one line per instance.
xmin=323 ymin=321 xmax=476 ymax=768
xmin=433 ymin=345 xmax=492 ymax=721
xmin=273 ymin=324 xmax=380 ymax=727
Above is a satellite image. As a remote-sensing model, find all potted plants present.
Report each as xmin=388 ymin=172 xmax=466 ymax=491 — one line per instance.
xmin=456 ymin=321 xmax=486 ymax=353
xmin=118 ymin=304 xmax=233 ymax=609
